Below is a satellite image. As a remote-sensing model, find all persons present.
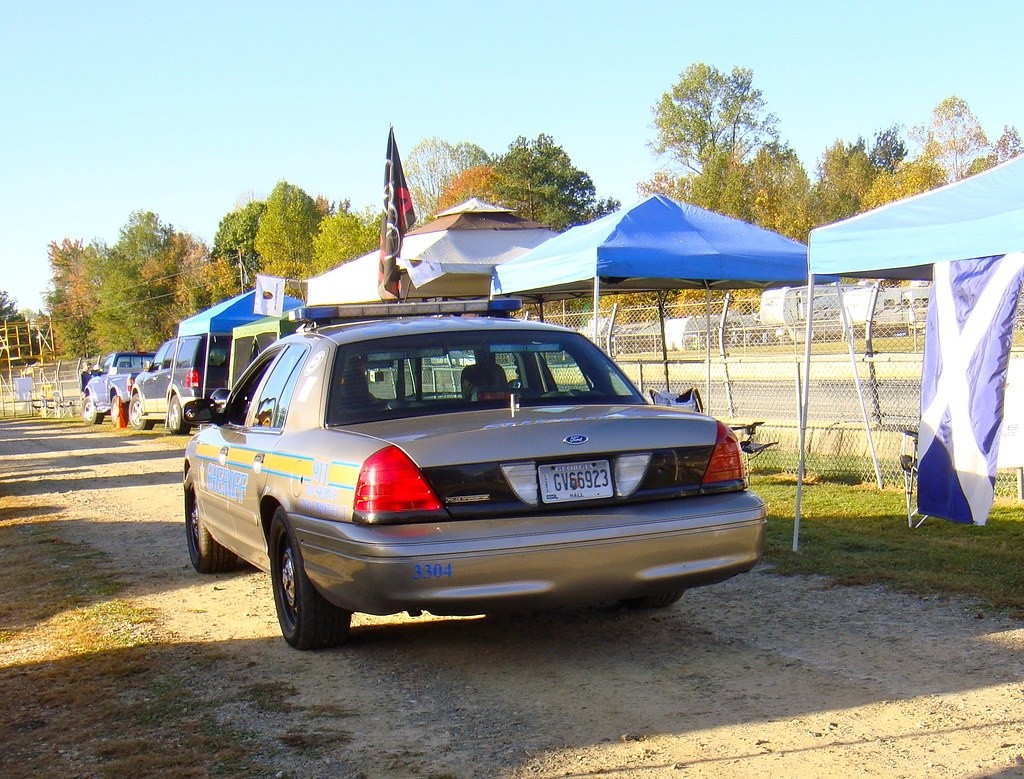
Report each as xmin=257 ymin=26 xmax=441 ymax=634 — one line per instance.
xmin=90 ymin=364 xmax=101 ymax=378
xmin=79 ymin=361 xmax=93 ymax=399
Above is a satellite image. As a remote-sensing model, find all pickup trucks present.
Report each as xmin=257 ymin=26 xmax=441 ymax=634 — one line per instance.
xmin=81 ymin=350 xmax=159 ymax=425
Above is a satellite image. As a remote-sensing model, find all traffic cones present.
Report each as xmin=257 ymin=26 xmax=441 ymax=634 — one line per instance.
xmin=114 ymin=397 xmax=127 ymax=430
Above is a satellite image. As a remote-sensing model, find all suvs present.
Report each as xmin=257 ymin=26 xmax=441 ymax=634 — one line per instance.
xmin=129 ymin=330 xmax=234 ymax=435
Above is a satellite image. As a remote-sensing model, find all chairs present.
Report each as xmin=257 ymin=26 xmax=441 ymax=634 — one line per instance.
xmin=461 ymin=363 xmax=508 ymax=401
xmin=651 ymin=386 xmax=780 ymax=485
xmin=902 ymin=429 xmax=930 ymax=528
xmin=341 ymin=370 xmax=369 ymax=410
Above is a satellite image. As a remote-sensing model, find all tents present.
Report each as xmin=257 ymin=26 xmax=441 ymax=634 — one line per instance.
xmin=163 ymin=289 xmax=305 ymax=440
xmin=227 ymin=304 xmax=307 ymax=391
xmin=489 ymin=192 xmax=885 ymax=493
xmin=792 ymin=151 xmax=1024 ymax=552
xmin=303 ymin=197 xmax=561 ymax=400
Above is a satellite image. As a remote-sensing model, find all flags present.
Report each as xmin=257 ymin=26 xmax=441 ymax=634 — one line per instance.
xmin=377 ymin=127 xmax=418 ymax=305
xmin=918 ymin=253 xmax=1024 ymax=526
xmin=253 ymin=276 xmax=286 ymax=317
xmin=396 ymin=258 xmax=447 ymax=289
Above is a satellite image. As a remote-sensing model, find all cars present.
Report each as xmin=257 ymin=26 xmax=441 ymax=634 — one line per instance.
xmin=185 ymin=304 xmax=768 ymax=653
